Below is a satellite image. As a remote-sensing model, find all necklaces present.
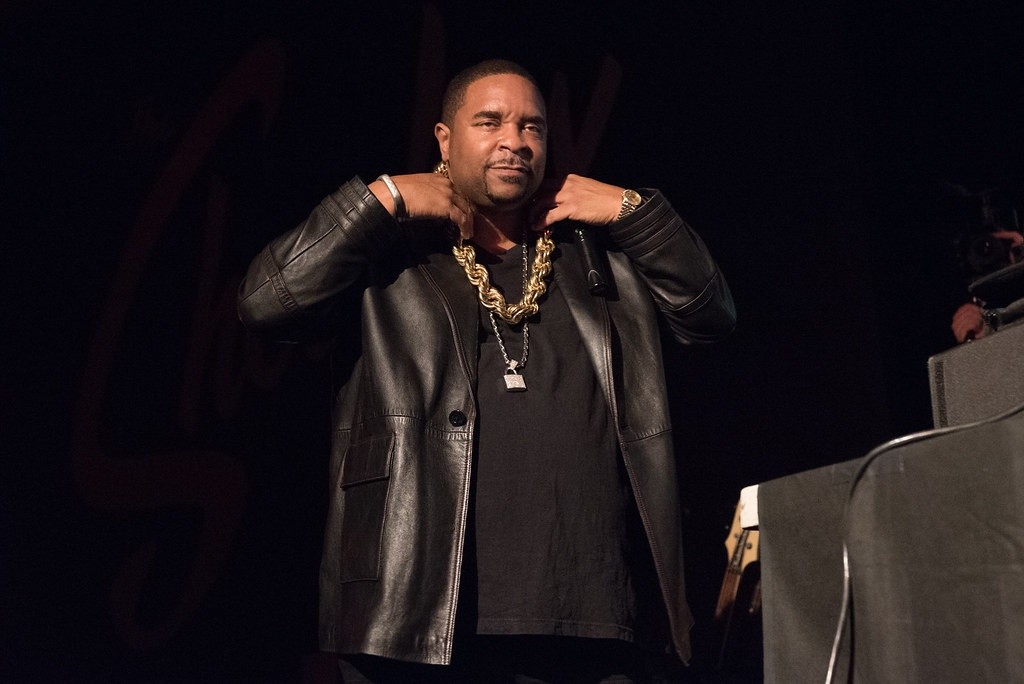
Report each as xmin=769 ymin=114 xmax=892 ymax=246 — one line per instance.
xmin=487 ymin=206 xmax=531 ymax=393
xmin=433 ymin=160 xmax=556 ymax=327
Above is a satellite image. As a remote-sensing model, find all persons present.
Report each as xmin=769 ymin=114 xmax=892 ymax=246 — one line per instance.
xmin=234 ymin=58 xmax=738 ymax=684
xmin=950 ymin=227 xmax=1022 ymax=343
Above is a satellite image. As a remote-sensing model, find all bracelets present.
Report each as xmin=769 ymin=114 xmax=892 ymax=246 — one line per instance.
xmin=376 ymin=173 xmax=405 ymax=219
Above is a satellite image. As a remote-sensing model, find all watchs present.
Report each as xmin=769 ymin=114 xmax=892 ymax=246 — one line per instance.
xmin=616 ymin=188 xmax=642 ymax=222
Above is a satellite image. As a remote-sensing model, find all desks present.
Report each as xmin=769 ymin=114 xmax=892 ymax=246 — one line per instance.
xmin=739 ymin=413 xmax=1024 ymax=684
